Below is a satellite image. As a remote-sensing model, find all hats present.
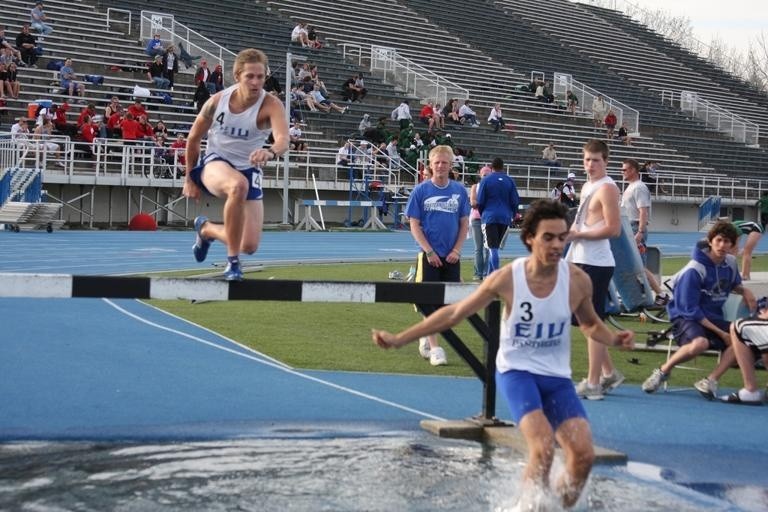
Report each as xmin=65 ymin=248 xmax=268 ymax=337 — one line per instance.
xmin=359 ymin=133 xmax=453 ymax=154
xmin=565 ymin=173 xmax=576 ymax=180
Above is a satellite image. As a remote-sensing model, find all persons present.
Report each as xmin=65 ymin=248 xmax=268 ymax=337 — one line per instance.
xmin=402 ymin=142 xmax=471 ymax=367
xmin=641 ymin=218 xmax=757 ymax=402
xmin=473 ymin=157 xmax=520 ymax=278
xmin=751 ymin=190 xmax=767 ymax=237
xmin=482 ymin=67 xmax=668 ymax=212
xmin=618 ymin=156 xmax=670 ymax=319
xmin=367 ymin=199 xmax=641 ymax=511
xmin=178 ymin=47 xmax=292 ymax=281
xmin=715 ymin=294 xmax=766 ymax=404
xmin=469 ymin=164 xmax=492 ymax=281
xmin=727 ymin=220 xmax=765 ymax=282
xmin=563 ymin=135 xmax=626 ymax=405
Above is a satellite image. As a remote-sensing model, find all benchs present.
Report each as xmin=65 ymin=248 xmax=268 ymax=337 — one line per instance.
xmin=0 ymin=0 xmax=768 ymax=200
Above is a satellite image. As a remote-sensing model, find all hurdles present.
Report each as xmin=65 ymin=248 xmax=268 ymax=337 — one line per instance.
xmin=0 ymin=168 xmax=66 ymax=231
xmin=294 ymin=200 xmax=388 ymax=232
xmin=0 ymin=271 xmax=516 ymax=429
xmin=697 ymin=195 xmax=722 ymax=233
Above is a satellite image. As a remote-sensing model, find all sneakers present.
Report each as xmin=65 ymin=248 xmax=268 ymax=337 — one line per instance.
xmin=429 ymin=121 xmax=511 ymax=132
xmin=718 ymin=384 xmax=764 ymax=407
xmin=694 ymin=376 xmax=720 ymax=400
xmin=190 ymin=215 xmax=214 ymax=262
xmin=54 ymin=162 xmax=65 ymax=167
xmin=223 ymin=253 xmax=243 ymax=282
xmin=574 ymin=377 xmax=604 ymax=403
xmin=309 ymin=105 xmax=350 ymax=115
xmin=641 ymin=366 xmax=669 ymax=394
xmin=388 ymin=270 xmax=404 ymax=279
xmin=599 ymin=368 xmax=627 ymax=395
xmin=417 ymin=333 xmax=449 ymax=366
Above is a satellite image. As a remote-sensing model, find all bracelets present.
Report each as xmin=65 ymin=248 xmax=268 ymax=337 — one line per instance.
xmin=264 ymin=146 xmax=277 ymax=160
xmin=451 ymin=248 xmax=461 ymax=257
xmin=426 ymin=249 xmax=435 ymax=258
